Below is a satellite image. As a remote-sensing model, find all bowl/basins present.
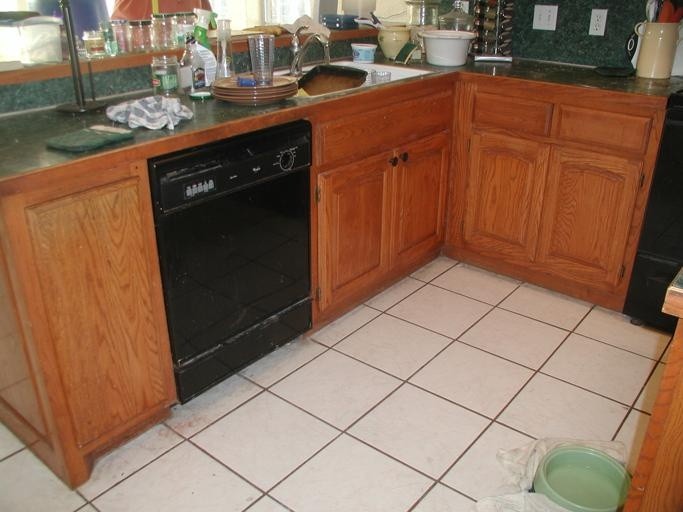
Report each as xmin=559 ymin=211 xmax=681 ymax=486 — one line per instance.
xmin=418 ymin=30 xmax=477 ymax=66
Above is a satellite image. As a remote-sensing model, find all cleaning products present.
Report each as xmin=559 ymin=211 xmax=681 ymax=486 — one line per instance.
xmin=192 ymin=7 xmax=218 ymax=88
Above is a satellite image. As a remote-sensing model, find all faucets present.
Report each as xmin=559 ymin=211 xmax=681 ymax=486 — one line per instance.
xmin=289 ymin=25 xmax=331 ymax=77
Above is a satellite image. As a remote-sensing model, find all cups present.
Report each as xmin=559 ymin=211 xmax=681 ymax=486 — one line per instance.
xmin=248 ymin=34 xmax=275 ymax=85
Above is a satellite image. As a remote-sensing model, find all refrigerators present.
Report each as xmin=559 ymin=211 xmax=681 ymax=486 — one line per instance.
xmin=148 ymin=117 xmax=313 ymax=405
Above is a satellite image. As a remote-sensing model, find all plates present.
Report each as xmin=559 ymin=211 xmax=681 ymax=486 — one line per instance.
xmin=210 ymin=75 xmax=298 ymax=105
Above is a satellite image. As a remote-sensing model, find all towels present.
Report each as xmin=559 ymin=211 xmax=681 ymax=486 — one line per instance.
xmin=105 ymin=94 xmax=194 ymax=131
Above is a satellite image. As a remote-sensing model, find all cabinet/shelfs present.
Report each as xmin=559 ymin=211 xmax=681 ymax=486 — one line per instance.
xmin=0 ymin=160 xmax=179 ymax=489
xmin=312 ymin=71 xmax=456 ymax=333
xmin=441 ymin=71 xmax=666 ymax=314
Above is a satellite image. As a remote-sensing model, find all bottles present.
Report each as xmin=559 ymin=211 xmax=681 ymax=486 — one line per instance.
xmin=180 ymin=32 xmax=206 ymax=96
xmin=405 ymin=0 xmax=437 ymax=61
xmin=215 ymin=19 xmax=235 ymax=80
xmin=82 ymin=11 xmax=198 ymax=59
xmin=150 ymin=55 xmax=182 ymax=101
xmin=439 ymin=1 xmax=475 ymax=31
